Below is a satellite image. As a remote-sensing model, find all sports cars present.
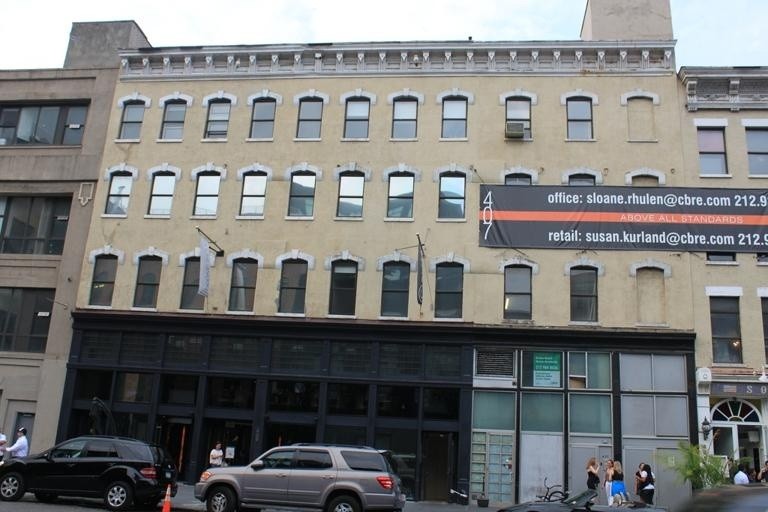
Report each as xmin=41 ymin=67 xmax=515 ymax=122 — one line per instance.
xmin=499 ymin=489 xmax=668 ymax=511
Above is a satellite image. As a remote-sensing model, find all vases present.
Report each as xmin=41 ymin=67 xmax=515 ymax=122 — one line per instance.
xmin=476 ymin=498 xmax=489 ymax=507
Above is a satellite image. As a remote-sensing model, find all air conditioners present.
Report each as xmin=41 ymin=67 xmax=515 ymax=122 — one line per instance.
xmin=506 ymin=123 xmax=524 ymax=138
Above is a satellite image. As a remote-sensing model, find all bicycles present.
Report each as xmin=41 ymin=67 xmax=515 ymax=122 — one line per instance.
xmin=536 ymin=478 xmax=565 ymax=501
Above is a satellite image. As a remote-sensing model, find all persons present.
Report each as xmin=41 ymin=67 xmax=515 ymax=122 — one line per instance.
xmin=728 ymin=457 xmax=768 ymax=484
xmin=0 ymin=432 xmax=7 ymax=462
xmin=210 ymin=442 xmax=224 ymax=467
xmin=0 ymin=427 xmax=28 ymax=458
xmin=586 ymin=458 xmax=655 ymax=506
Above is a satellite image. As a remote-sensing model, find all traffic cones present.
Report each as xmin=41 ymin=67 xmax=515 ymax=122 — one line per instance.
xmin=162 ymin=484 xmax=172 ymax=512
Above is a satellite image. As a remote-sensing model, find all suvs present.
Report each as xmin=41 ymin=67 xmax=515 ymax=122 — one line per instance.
xmin=0 ymin=435 xmax=177 ymax=512
xmin=194 ymin=442 xmax=405 ymax=512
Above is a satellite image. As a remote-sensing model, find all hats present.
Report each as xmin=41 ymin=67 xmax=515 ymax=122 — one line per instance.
xmin=16 ymin=428 xmax=27 ymax=434
